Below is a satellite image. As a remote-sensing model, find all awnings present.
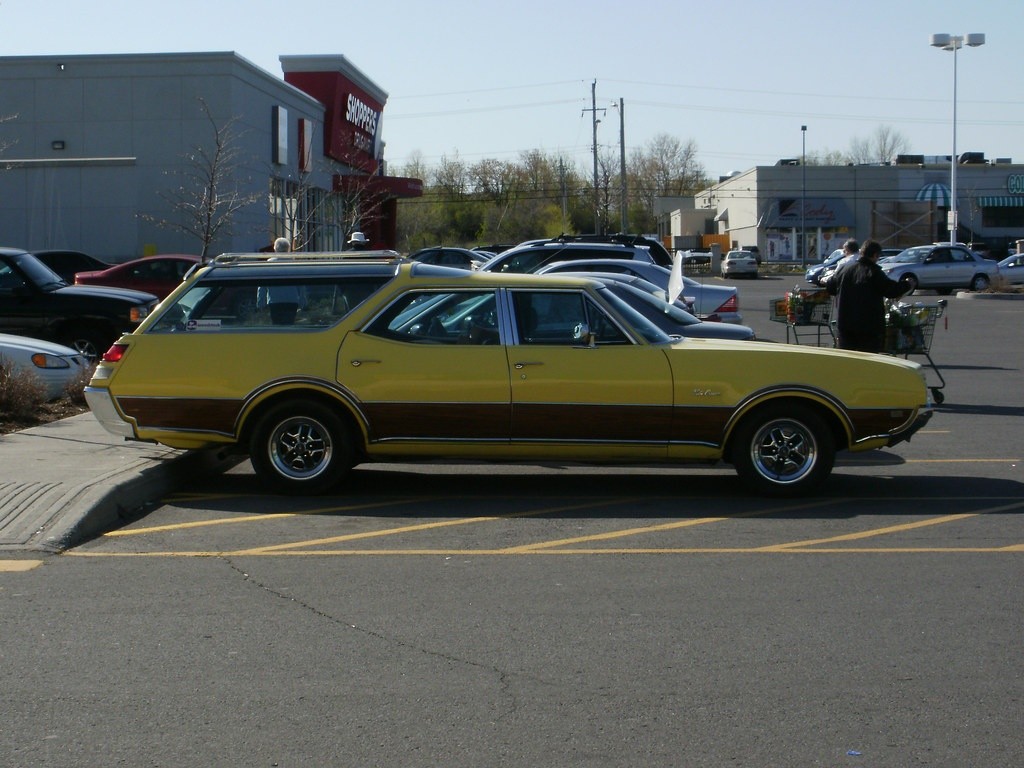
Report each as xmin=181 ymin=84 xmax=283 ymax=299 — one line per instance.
xmin=756 ymin=197 xmax=859 ymax=228
xmin=713 ymin=208 xmax=728 ymax=221
xmin=913 ymin=182 xmax=961 ymax=207
xmin=976 ymin=196 xmax=1024 ymax=207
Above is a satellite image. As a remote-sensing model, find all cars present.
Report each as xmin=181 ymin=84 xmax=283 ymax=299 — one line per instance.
xmin=420 ymin=259 xmax=755 ymax=343
xmin=877 ymin=245 xmax=999 ymax=294
xmin=405 ymin=244 xmax=512 ymax=273
xmin=0 ymin=251 xmax=114 ymax=290
xmin=720 ymin=250 xmax=757 ymax=277
xmin=0 ymin=333 xmax=90 ymax=402
xmin=805 ymin=250 xmax=896 ymax=287
xmin=74 ymin=255 xmax=212 ymax=303
xmin=997 ymin=252 xmax=1024 ymax=286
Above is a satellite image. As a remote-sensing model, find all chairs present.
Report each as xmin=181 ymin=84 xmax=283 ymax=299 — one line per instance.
xmin=347 ymin=278 xmax=384 ymax=310
xmin=519 ymin=307 xmax=537 ymax=337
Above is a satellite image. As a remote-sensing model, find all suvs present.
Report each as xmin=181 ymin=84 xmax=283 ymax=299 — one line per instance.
xmin=476 ymin=239 xmax=657 ymax=279
xmin=514 ymin=233 xmax=675 ymax=270
xmin=0 ymin=246 xmax=161 ymax=367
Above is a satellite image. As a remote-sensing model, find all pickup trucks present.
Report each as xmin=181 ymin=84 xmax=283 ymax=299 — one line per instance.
xmin=83 ymin=249 xmax=936 ymax=502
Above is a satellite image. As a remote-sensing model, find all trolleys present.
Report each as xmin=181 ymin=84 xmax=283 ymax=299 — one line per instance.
xmin=769 ymin=284 xmax=839 ymax=348
xmin=884 ymin=295 xmax=948 ymax=405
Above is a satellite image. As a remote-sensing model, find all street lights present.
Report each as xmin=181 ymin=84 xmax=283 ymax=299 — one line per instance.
xmin=930 ymin=33 xmax=986 ymax=245
xmin=801 ymin=125 xmax=808 ymax=269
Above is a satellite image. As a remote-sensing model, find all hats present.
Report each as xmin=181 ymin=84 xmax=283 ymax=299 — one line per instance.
xmin=347 ymin=231 xmax=370 ymax=244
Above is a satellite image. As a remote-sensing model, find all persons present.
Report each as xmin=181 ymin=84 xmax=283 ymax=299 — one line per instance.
xmin=336 ymin=232 xmax=370 ymax=312
xmin=768 ymin=241 xmax=774 ymax=258
xmin=257 ymin=237 xmax=308 ymax=326
xmin=784 ymin=237 xmax=790 ymax=253
xmin=820 ymin=237 xmax=916 ymax=353
